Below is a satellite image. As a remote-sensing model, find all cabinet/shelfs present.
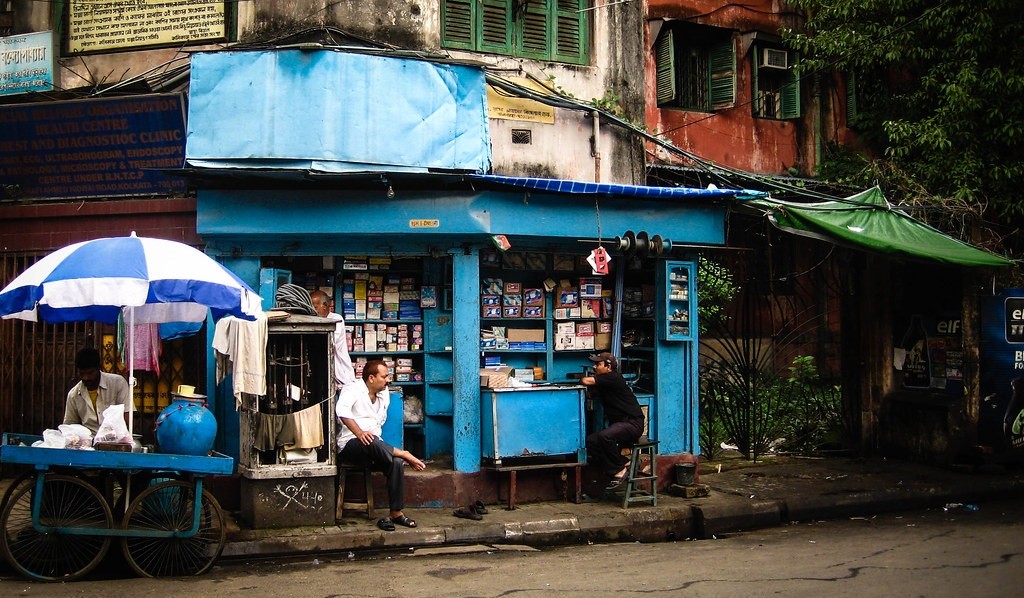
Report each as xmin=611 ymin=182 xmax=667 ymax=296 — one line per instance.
xmin=476 ymin=246 xmax=552 ymax=388
xmin=548 ymin=243 xmax=623 ymax=386
xmin=335 ymin=254 xmax=429 ymax=462
xmin=659 ymin=258 xmax=696 ymax=342
xmin=623 ymin=254 xmax=657 ymax=393
xmin=424 ymin=347 xmax=455 ymax=417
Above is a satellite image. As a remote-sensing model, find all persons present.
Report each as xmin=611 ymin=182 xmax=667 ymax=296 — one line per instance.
xmin=309 ymin=290 xmax=356 ymax=429
xmin=580 ymin=353 xmax=646 ymax=490
xmin=63 ymin=348 xmax=144 ymax=520
xmin=335 ymin=359 xmax=425 ymax=527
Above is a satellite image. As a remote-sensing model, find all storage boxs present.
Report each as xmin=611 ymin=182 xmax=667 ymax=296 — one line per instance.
xmin=506 ymin=327 xmax=545 ymax=342
xmin=553 ymin=277 xmax=610 ymax=351
xmin=478 ymin=365 xmax=513 ymax=389
xmin=340 ymin=257 xmax=422 ymax=383
xmin=480 ymin=276 xmax=545 ymax=319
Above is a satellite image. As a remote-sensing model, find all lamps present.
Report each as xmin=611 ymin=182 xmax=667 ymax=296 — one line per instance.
xmin=386 ymin=179 xmax=395 ymax=199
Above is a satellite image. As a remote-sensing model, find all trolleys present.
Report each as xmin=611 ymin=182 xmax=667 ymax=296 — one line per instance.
xmin=0 ymin=430 xmax=235 ymax=580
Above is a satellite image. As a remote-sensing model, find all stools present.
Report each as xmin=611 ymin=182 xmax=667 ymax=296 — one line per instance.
xmin=606 ymin=437 xmax=660 ymax=509
xmin=335 ymin=459 xmax=377 ymax=520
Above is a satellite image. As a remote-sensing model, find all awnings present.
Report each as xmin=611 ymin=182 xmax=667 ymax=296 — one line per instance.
xmin=739 ymin=184 xmax=1018 ymax=266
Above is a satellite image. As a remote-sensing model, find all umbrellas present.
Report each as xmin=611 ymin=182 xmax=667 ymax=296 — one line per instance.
xmin=0 ymin=231 xmax=264 ymax=514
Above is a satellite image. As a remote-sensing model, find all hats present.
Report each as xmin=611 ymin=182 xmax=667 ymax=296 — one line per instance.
xmin=588 ymin=352 xmax=618 ymax=365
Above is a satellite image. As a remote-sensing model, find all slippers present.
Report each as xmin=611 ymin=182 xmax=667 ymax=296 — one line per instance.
xmin=605 ymin=460 xmax=631 ymax=489
xmin=453 ymin=504 xmax=482 ymax=520
xmin=390 ymin=513 xmax=418 ymax=527
xmin=471 ymin=500 xmax=489 ymax=513
xmin=377 ymin=517 xmax=394 ymax=531
